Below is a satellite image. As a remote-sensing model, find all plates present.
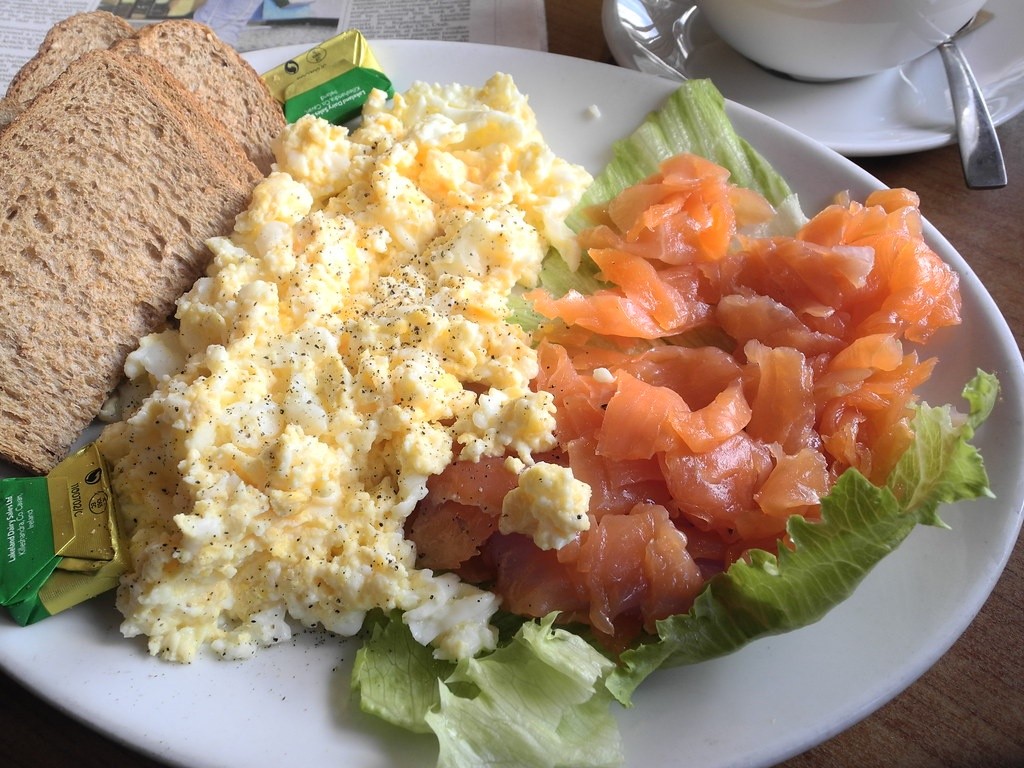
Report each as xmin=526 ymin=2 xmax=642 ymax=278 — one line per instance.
xmin=600 ymin=1 xmax=1024 ymax=159
xmin=0 ymin=39 xmax=1024 ymax=768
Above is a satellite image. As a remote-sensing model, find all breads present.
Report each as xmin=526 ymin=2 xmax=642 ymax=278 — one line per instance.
xmin=4 ymin=9 xmax=290 ymax=475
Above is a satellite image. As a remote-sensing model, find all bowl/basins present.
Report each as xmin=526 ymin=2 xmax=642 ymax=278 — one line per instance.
xmin=696 ymin=0 xmax=988 ymax=82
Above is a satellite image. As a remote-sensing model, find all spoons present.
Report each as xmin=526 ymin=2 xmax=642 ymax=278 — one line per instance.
xmin=937 ymin=14 xmax=1014 ymax=192
